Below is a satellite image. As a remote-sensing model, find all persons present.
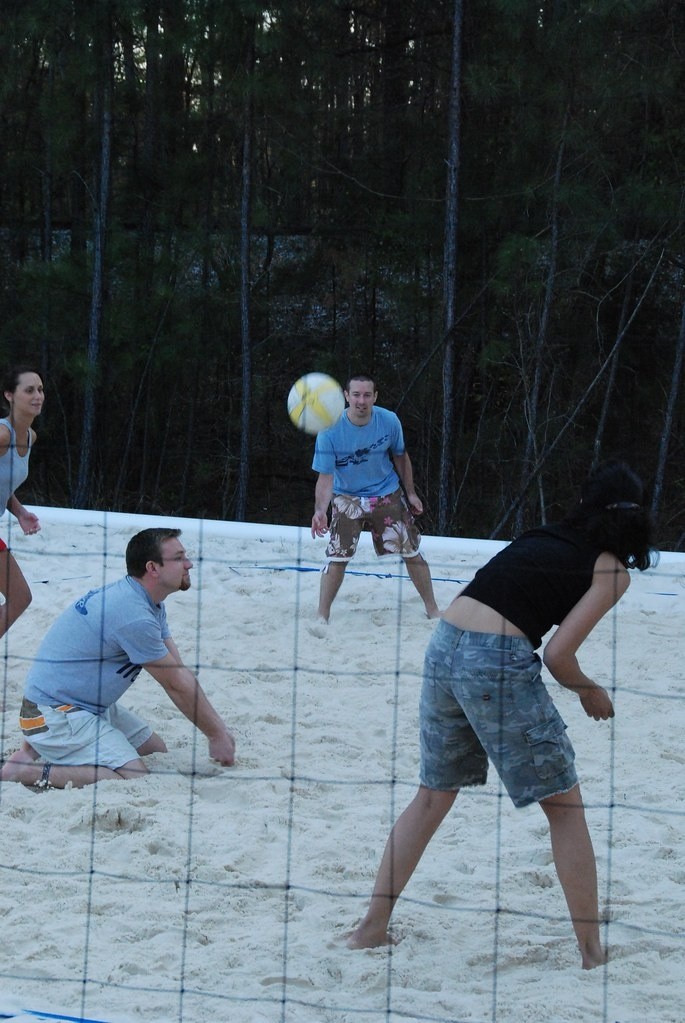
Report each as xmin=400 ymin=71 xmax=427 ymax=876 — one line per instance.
xmin=311 ymin=373 xmax=441 ymax=624
xmin=0 ymin=365 xmax=45 ymax=641
xmin=0 ymin=527 xmax=236 ymax=789
xmin=351 ymin=461 xmax=655 ymax=970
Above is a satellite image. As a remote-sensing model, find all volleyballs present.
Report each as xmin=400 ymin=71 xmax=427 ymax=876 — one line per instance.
xmin=289 ymin=371 xmax=343 ymax=433
